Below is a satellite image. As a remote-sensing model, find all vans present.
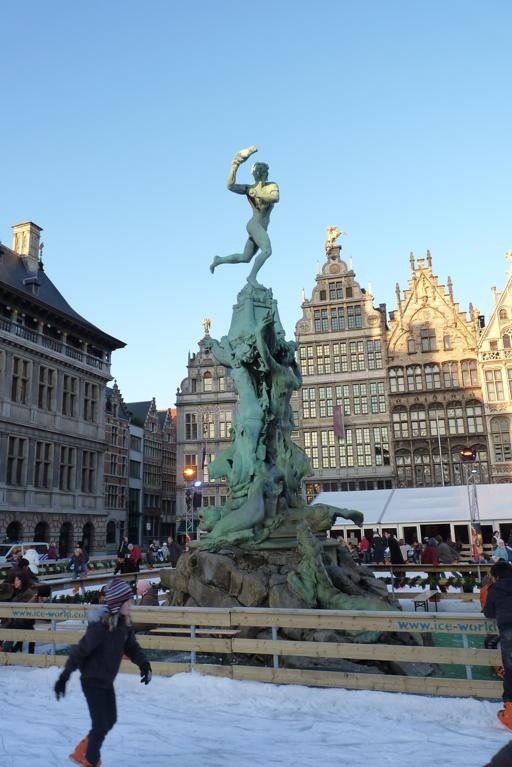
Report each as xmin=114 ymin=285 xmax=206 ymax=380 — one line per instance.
xmin=1 ymin=539 xmax=52 ymax=576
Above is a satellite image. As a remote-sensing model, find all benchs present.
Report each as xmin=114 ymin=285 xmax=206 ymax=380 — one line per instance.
xmin=411 ymin=589 xmax=438 ymax=612
xmin=149 ymin=627 xmax=240 ymax=660
xmin=357 ymin=540 xmax=498 ymax=565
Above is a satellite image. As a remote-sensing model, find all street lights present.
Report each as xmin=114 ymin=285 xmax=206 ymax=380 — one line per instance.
xmin=183 ymin=466 xmax=202 ymax=540
xmin=459 ymin=447 xmax=485 ymax=562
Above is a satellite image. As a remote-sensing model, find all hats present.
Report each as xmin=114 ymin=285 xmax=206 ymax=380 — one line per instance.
xmin=105 ymin=578 xmax=134 ymax=615
xmin=137 ymin=579 xmax=155 ymax=595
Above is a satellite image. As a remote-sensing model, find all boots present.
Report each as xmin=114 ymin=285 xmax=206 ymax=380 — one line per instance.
xmin=498 ymin=701 xmax=512 ymax=730
xmin=76 ymin=736 xmax=102 ymax=767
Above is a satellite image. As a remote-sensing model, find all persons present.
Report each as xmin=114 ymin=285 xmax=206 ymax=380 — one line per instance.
xmin=490 ymin=530 xmax=500 ymax=550
xmin=336 ymin=522 xmax=463 ymax=591
xmin=255 ymin=310 xmax=304 ymax=432
xmin=1 ymin=541 xmax=58 ymax=663
xmin=209 ymin=142 xmax=280 ymax=292
xmin=53 ymin=577 xmax=155 ymax=766
xmin=75 ymin=540 xmax=90 ymax=574
xmin=65 ymin=548 xmax=86 ymax=583
xmin=481 ymin=560 xmax=512 ymax=732
xmin=135 ymin=579 xmax=161 ymax=605
xmin=492 ymin=538 xmax=508 ymax=563
xmin=89 ymin=584 xmax=107 ymax=605
xmin=115 ymin=531 xmax=184 ymax=577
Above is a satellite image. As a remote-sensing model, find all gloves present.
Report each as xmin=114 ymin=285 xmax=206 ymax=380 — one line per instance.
xmin=55 ymin=672 xmax=69 ymax=700
xmin=140 ymin=662 xmax=152 ymax=685
